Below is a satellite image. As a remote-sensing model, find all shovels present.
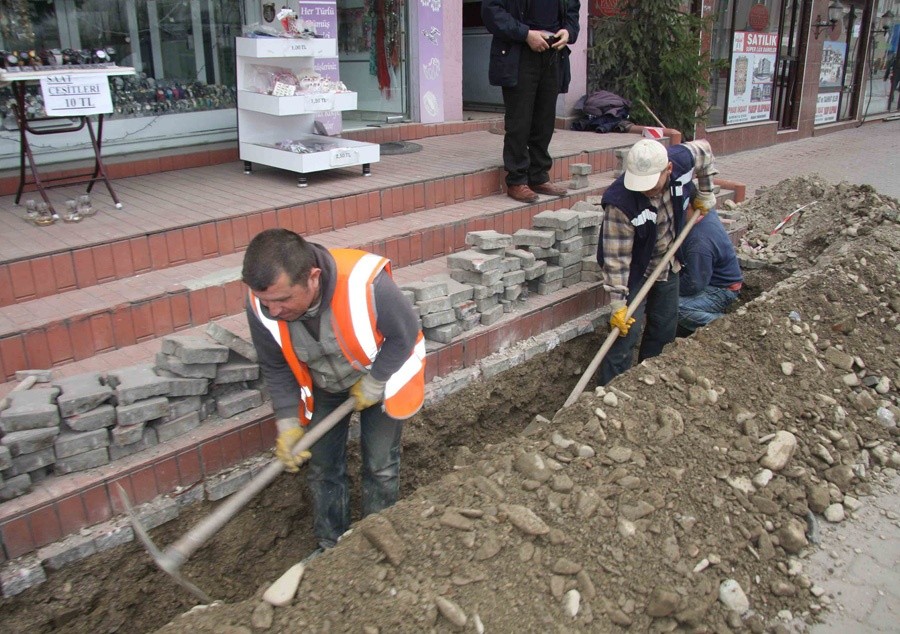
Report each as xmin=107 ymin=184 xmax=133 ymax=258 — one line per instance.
xmin=519 ymin=207 xmax=703 ymax=435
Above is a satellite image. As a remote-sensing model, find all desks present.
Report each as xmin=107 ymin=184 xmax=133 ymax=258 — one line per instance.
xmin=0 ymin=66 xmax=136 ymax=219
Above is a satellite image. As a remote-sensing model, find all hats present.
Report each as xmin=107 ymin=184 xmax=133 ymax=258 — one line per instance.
xmin=624 ymin=140 xmax=668 ymax=191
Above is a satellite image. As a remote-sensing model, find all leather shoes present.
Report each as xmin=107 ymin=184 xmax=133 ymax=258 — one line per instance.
xmin=506 ymin=185 xmax=539 ymax=202
xmin=530 ymin=182 xmax=567 ymax=196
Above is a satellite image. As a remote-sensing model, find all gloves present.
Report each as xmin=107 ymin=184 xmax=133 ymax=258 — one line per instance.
xmin=277 ymin=418 xmax=312 ymax=473
xmin=694 ymin=190 xmax=716 ymax=215
xmin=349 ymin=372 xmax=386 ymax=411
xmin=610 ymin=307 xmax=636 ymax=337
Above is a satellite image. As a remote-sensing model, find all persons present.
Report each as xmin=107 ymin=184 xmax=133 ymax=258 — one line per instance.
xmin=678 ymin=181 xmax=744 ymax=332
xmin=241 ymin=226 xmax=426 ymax=553
xmin=597 ymin=139 xmax=719 ymax=386
xmin=480 ymin=0 xmax=581 ymax=202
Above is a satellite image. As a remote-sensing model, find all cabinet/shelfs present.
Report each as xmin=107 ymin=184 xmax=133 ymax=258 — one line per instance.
xmin=235 ymin=36 xmax=381 ymax=188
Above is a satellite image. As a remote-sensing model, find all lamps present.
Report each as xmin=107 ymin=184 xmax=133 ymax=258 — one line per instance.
xmin=871 ymin=10 xmax=895 ymax=37
xmin=811 ymin=0 xmax=844 ymax=40
